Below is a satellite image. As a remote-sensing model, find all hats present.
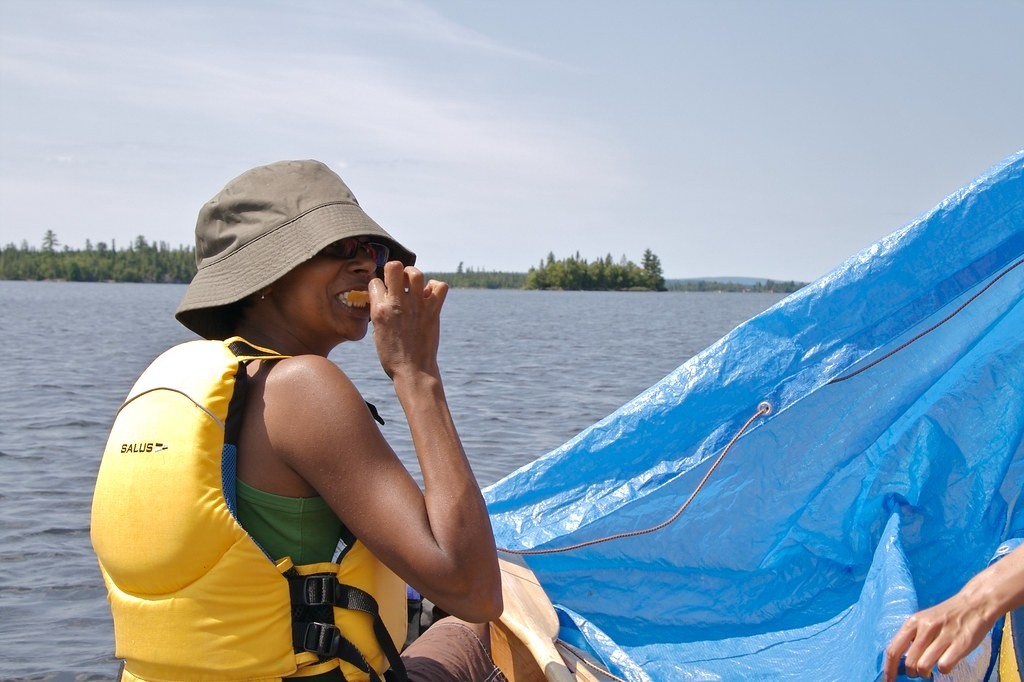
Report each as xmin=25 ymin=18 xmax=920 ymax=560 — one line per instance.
xmin=172 ymin=157 xmax=417 ymax=340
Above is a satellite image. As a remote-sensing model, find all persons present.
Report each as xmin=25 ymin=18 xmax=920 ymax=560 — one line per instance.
xmin=883 ymin=541 xmax=1024 ymax=682
xmin=87 ymin=158 xmax=513 ymax=682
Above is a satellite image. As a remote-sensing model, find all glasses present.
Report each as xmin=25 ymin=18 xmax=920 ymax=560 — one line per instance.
xmin=320 ymin=237 xmax=390 ymax=266
